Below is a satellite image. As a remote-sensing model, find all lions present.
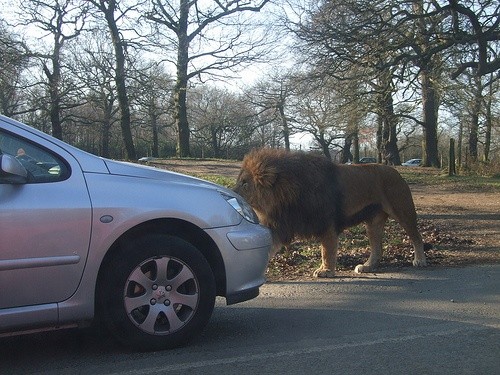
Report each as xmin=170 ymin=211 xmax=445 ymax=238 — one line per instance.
xmin=233 ymin=146 xmax=426 ymax=278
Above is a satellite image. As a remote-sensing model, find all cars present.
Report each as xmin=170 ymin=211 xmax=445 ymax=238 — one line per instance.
xmin=345 ymin=156 xmax=377 ymax=165
xmin=1 ymin=111 xmax=273 ymax=353
xmin=400 ymin=158 xmax=423 ymax=167
